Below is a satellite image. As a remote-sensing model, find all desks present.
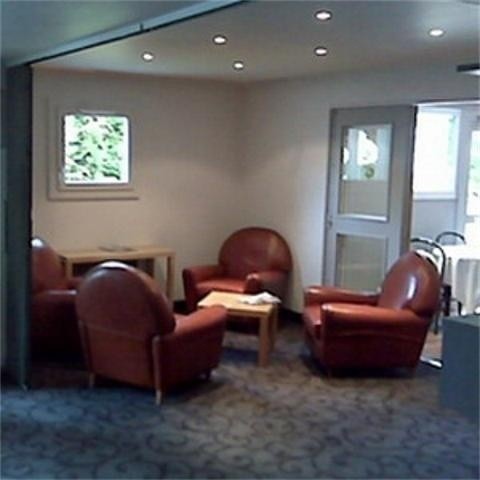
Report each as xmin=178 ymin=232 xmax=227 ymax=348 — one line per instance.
xmin=433 ymin=245 xmax=480 ymax=318
xmin=54 ymin=245 xmax=176 ymax=305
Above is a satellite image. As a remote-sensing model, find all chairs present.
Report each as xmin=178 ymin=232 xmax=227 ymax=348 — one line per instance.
xmin=434 ymin=232 xmax=466 ymax=316
xmin=410 ymin=237 xmax=452 ymax=334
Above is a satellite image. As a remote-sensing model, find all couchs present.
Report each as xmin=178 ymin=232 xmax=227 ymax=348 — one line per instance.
xmin=303 ymin=251 xmax=440 ymax=379
xmin=183 ymin=229 xmax=291 ymax=336
xmin=28 ymin=237 xmax=87 ymax=386
xmin=74 ymin=262 xmax=228 ymax=405
